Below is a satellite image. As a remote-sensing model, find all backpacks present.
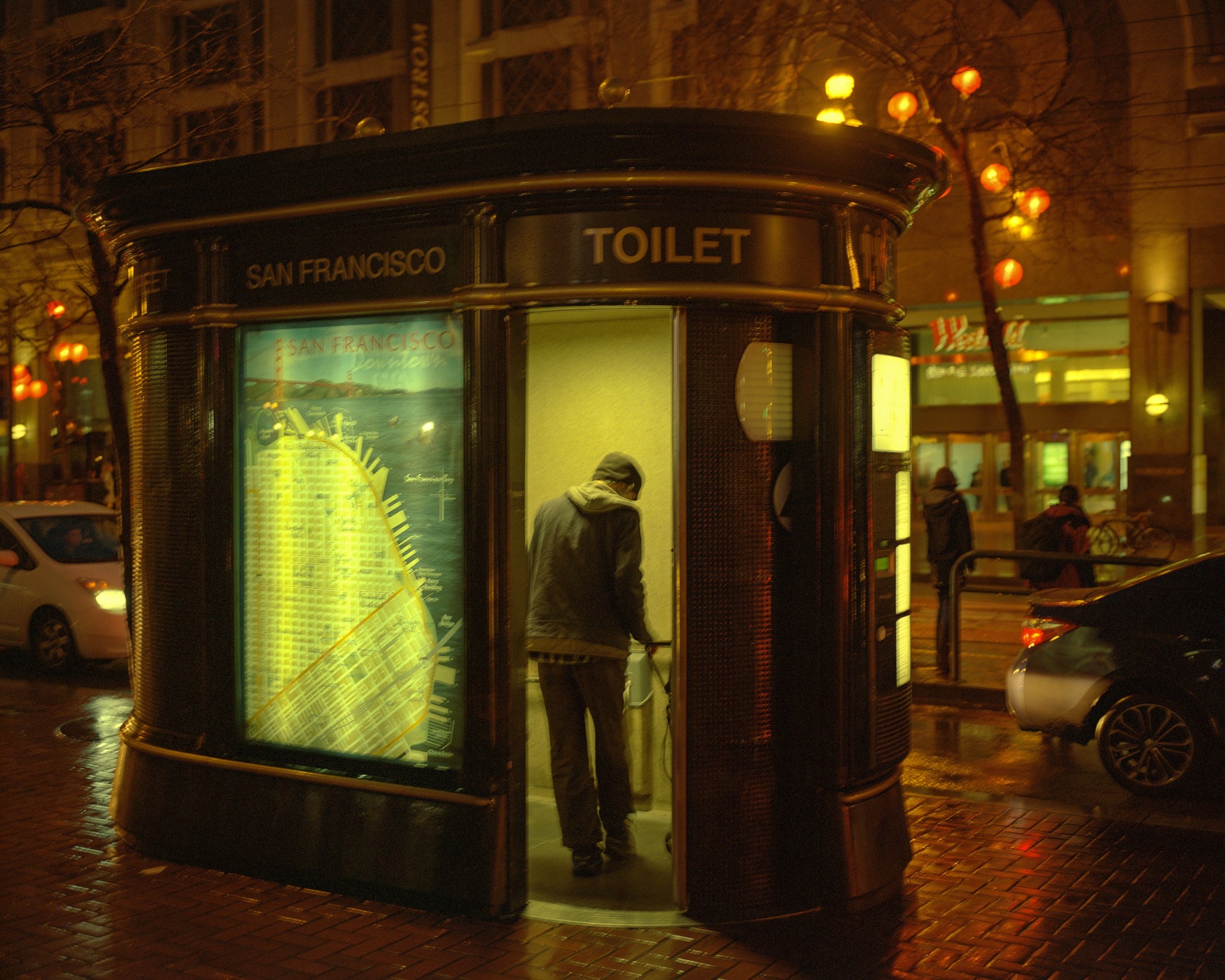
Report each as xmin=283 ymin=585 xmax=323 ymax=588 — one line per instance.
xmin=1020 ymin=513 xmax=1073 ymax=580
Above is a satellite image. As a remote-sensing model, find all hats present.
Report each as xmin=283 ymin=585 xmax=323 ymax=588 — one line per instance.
xmin=593 ymin=454 xmax=641 ymax=501
xmin=933 ymin=467 xmax=956 ymax=486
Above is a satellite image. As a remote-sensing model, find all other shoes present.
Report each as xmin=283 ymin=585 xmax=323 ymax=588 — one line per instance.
xmin=605 ymin=826 xmax=633 ymax=857
xmin=571 ymin=847 xmax=604 ymax=875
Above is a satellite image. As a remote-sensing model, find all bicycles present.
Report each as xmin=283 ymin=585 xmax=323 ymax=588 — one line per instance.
xmin=1086 ymin=506 xmax=1175 ymax=562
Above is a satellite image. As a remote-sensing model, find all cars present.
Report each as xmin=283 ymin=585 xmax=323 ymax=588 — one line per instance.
xmin=0 ymin=500 xmax=130 ymax=678
xmin=1005 ymin=548 xmax=1225 ymax=796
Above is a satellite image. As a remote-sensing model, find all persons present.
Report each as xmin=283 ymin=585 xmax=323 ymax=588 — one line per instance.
xmin=1035 ymin=483 xmax=1095 ymax=591
xmin=1000 ymin=460 xmax=1013 ymax=509
xmin=925 ymin=466 xmax=974 ymax=678
xmin=44 ymin=525 xmax=99 ymax=566
xmin=526 ymin=450 xmax=655 ymax=872
xmin=971 ymin=463 xmax=983 ymax=510
